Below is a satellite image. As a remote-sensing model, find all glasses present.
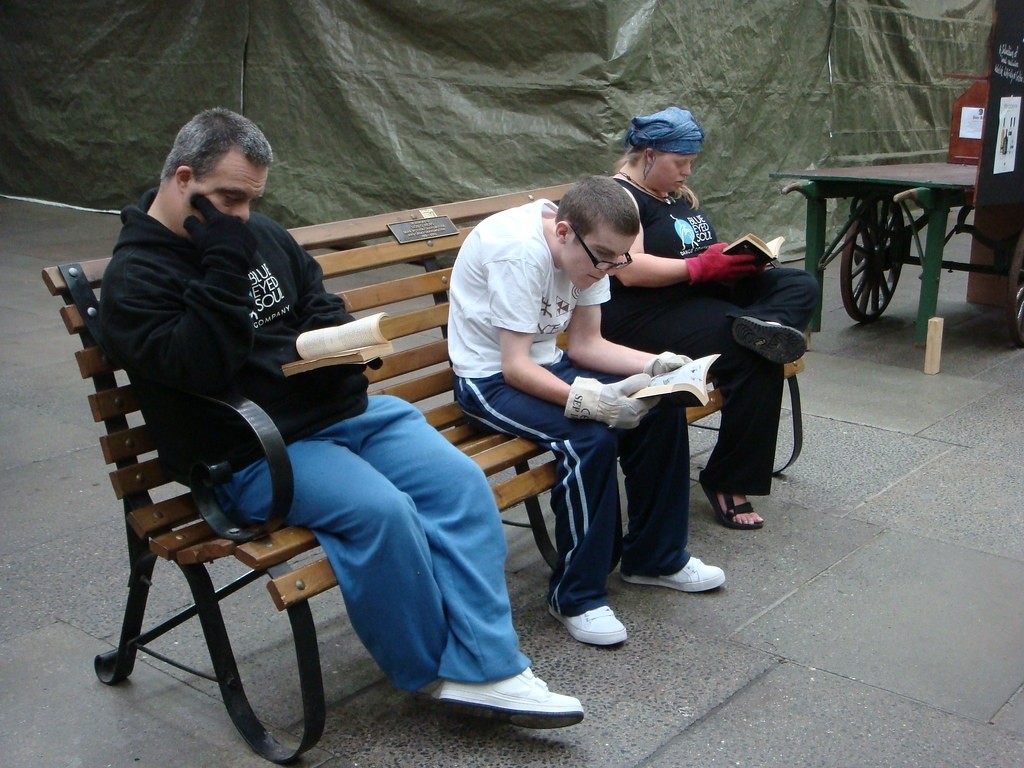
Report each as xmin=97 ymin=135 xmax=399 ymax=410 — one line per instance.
xmin=568 ymin=224 xmax=633 ymax=272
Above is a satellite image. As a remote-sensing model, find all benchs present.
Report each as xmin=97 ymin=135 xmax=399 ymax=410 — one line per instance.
xmin=39 ymin=181 xmax=803 ymax=766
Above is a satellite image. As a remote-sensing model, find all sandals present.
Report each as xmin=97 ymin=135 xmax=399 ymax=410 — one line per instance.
xmin=731 ymin=316 xmax=808 ymax=364
xmin=698 ymin=469 xmax=764 ymax=529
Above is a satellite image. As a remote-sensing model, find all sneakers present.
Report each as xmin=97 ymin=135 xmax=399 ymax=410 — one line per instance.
xmin=620 ymin=556 xmax=726 ymax=592
xmin=548 ymin=603 xmax=628 ymax=645
xmin=437 ymin=666 xmax=585 ymax=728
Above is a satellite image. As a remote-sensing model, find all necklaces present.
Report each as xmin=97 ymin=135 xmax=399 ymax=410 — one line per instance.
xmin=616 ymin=172 xmax=671 ymax=205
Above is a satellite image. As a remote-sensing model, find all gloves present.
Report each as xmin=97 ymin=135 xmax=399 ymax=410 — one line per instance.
xmin=684 ymin=242 xmax=767 ymax=287
xmin=642 ymin=351 xmax=694 ymax=378
xmin=563 ymin=373 xmax=662 ymax=430
xmin=182 ymin=191 xmax=259 ymax=276
xmin=294 ymin=313 xmax=384 ymax=378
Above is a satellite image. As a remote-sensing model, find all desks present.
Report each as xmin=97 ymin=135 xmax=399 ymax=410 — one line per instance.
xmin=769 ymin=160 xmax=978 ymax=349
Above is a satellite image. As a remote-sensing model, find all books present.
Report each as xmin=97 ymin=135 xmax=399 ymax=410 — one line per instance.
xmin=283 ymin=312 xmax=393 ymax=378
xmin=628 ymin=354 xmax=721 ymax=407
xmin=722 ymin=233 xmax=785 ymax=267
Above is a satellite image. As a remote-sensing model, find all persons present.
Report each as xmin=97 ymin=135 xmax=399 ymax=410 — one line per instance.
xmin=600 ymin=106 xmax=820 ymax=529
xmin=101 ymin=109 xmax=587 ymax=730
xmin=447 ymin=176 xmax=725 ymax=646
